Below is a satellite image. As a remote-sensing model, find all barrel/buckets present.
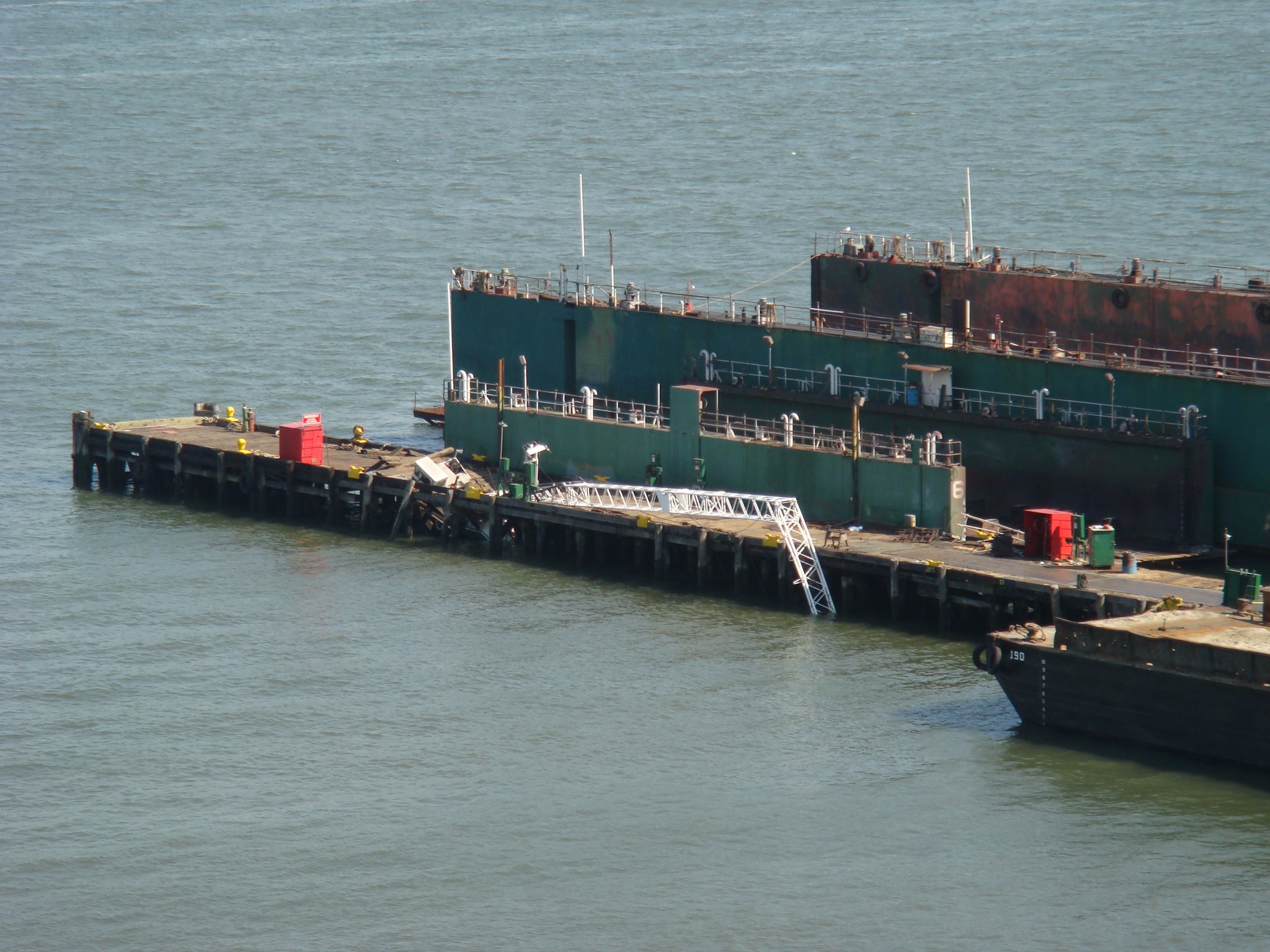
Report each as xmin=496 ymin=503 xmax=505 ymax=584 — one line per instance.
xmin=907 ymin=388 xmax=920 ymax=407
xmin=1122 ymin=552 xmax=1137 ymax=574
xmin=904 ymin=514 xmax=916 ymax=528
